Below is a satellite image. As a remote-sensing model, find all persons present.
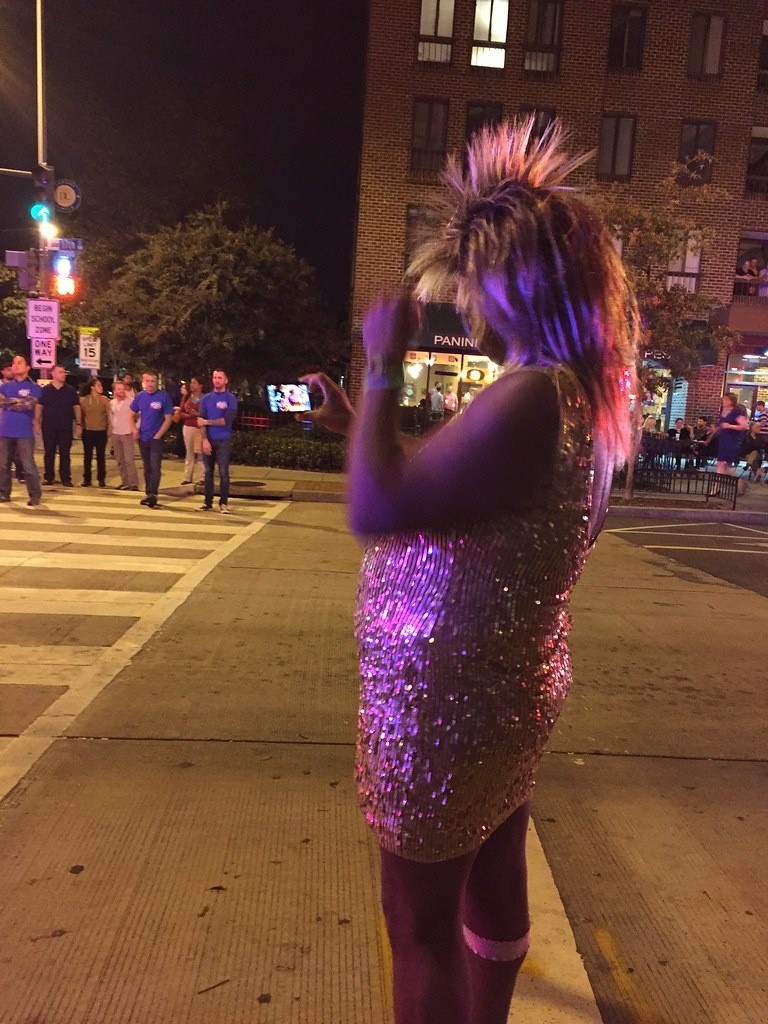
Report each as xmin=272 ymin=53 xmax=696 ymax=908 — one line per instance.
xmin=0 ymin=354 xmax=44 ymax=506
xmin=419 ymin=381 xmax=472 ymax=420
xmin=32 ymin=365 xmax=82 ymax=488
xmin=128 ymin=371 xmax=173 ymax=507
xmin=636 ymin=392 xmax=768 ymax=497
xmin=268 ymin=384 xmax=301 ymax=413
xmin=174 ymin=378 xmax=206 ymax=485
xmin=196 ymin=368 xmax=237 ymax=514
xmin=106 ymin=381 xmax=141 ymax=492
xmin=295 ymin=176 xmax=645 ymax=1024
xmin=109 ymin=373 xmax=138 ymax=456
xmin=79 ymin=378 xmax=110 ymax=487
xmin=734 ymin=258 xmax=768 ymax=296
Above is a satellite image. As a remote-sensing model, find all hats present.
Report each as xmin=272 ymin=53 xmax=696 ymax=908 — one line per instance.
xmin=754 ymin=401 xmax=765 ymax=407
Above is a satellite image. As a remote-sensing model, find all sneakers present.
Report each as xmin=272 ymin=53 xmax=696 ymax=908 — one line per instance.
xmin=195 ymin=504 xmax=213 ymax=511
xmin=220 ymin=503 xmax=230 ymax=513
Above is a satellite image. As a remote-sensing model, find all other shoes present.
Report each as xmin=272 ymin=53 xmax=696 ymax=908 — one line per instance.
xmin=131 ymin=486 xmax=138 ymax=491
xmin=99 ymin=480 xmax=105 ymax=486
xmin=19 ymin=476 xmax=25 ymax=482
xmin=141 ymin=495 xmax=157 ymax=507
xmin=42 ymin=479 xmax=51 ymax=484
xmin=63 ymin=480 xmax=73 ymax=487
xmin=0 ymin=498 xmax=9 ymax=502
xmin=181 ymin=480 xmax=192 ymax=485
xmin=80 ymin=479 xmax=90 ymax=486
xmin=27 ymin=499 xmax=36 ymax=506
xmin=197 ymin=481 xmax=205 ymax=485
xmin=116 ymin=484 xmax=128 ymax=490
xmin=673 ymin=464 xmax=680 ymax=471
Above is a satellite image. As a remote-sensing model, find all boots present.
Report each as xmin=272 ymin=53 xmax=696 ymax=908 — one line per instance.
xmin=743 ymin=463 xmax=750 ymax=471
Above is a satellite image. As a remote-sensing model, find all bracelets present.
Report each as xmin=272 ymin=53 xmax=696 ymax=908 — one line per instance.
xmin=76 ymin=423 xmax=82 ymax=427
xmin=363 ymin=375 xmax=401 ymax=393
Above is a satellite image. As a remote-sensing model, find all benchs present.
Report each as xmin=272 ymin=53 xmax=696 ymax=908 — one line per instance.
xmin=639 ymin=439 xmax=715 ymax=461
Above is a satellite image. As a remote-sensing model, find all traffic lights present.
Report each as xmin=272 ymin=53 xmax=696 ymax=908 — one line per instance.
xmin=51 ymin=251 xmax=75 ymax=298
xmin=32 ymin=163 xmax=55 ymax=225
xmin=5 ymin=251 xmax=35 ymax=291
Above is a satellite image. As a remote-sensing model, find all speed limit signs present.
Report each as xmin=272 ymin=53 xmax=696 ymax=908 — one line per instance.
xmin=79 ymin=336 xmax=100 ymax=369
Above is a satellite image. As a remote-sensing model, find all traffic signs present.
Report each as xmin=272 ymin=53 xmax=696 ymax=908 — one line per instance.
xmin=31 ymin=338 xmax=56 ymax=371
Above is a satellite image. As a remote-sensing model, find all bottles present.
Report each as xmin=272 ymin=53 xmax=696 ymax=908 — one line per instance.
xmin=674 ymin=429 xmax=681 ymax=441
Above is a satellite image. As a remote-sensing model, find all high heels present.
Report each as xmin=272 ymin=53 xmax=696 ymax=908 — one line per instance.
xmin=706 ymin=490 xmax=721 ymax=497
xmin=738 ymin=483 xmax=749 ymax=497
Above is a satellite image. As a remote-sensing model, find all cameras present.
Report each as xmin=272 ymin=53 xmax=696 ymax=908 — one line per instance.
xmin=263 ymin=383 xmax=313 ymax=417
